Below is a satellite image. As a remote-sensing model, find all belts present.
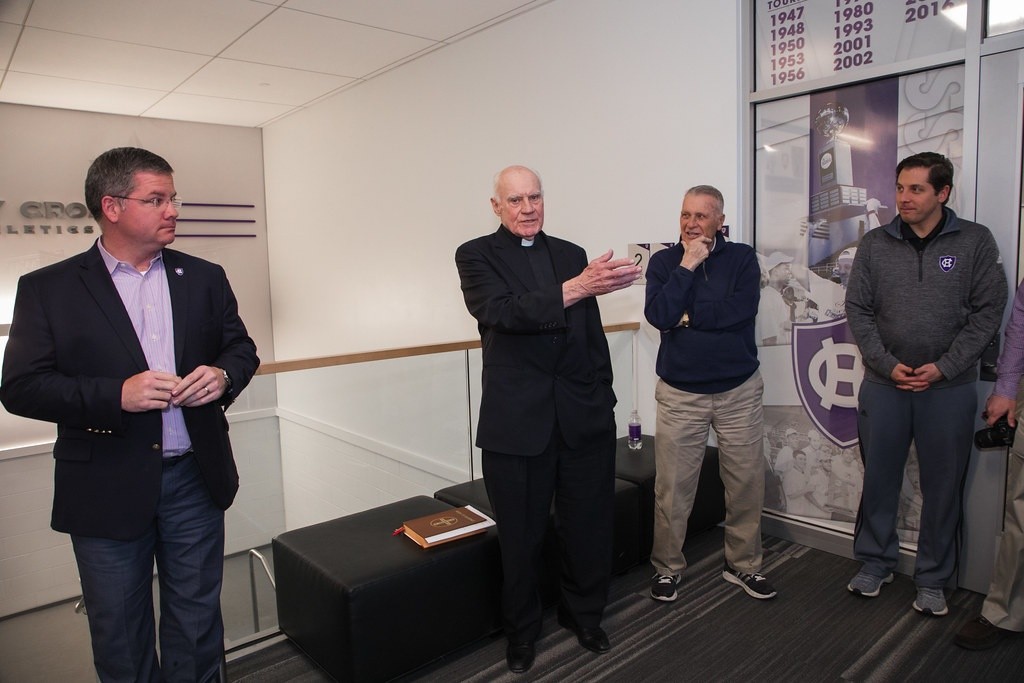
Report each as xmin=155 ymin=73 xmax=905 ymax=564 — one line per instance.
xmin=162 ymin=449 xmax=195 ymax=468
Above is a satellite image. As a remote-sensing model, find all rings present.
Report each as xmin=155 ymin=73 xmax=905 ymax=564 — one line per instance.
xmin=204 ymin=387 xmax=209 ymax=394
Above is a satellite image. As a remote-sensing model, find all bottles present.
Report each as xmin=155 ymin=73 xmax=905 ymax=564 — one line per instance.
xmin=628 ymin=410 xmax=643 ymax=449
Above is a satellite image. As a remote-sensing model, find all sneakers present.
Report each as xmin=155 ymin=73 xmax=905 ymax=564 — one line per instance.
xmin=846 ymin=563 xmax=893 ymax=597
xmin=953 ymin=614 xmax=1010 ymax=650
xmin=650 ymin=572 xmax=681 ymax=602
xmin=912 ymin=586 xmax=950 ymax=618
xmin=722 ymin=557 xmax=777 ymax=599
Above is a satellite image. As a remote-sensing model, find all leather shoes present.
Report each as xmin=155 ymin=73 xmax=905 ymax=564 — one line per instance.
xmin=505 ymin=642 xmax=536 ymax=673
xmin=575 ymin=626 xmax=610 ymax=654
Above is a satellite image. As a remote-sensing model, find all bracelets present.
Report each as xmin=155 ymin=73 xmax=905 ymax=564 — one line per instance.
xmin=682 ymin=311 xmax=689 ymax=327
xmin=867 ymin=209 xmax=878 ymax=219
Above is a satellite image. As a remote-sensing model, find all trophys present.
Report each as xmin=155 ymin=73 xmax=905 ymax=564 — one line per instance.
xmin=808 ymin=103 xmax=868 ymax=223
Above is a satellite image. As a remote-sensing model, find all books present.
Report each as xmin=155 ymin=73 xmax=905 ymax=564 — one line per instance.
xmin=403 ymin=507 xmax=488 ymax=548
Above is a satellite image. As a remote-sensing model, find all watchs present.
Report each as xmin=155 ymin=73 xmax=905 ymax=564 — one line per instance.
xmin=220 ymin=368 xmax=233 ymax=385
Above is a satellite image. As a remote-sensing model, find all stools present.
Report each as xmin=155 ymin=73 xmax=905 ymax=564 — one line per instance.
xmin=433 ymin=478 xmax=639 ymax=610
xmin=271 ymin=495 xmax=506 ymax=683
xmin=615 ymin=434 xmax=727 ymax=563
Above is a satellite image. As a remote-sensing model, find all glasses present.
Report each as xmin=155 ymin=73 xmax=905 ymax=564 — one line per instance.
xmin=110 ymin=195 xmax=182 ymax=210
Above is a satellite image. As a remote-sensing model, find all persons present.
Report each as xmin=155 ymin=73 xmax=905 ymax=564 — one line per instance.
xmin=754 ymin=199 xmax=881 ymax=345
xmin=455 ymin=166 xmax=642 ymax=673
xmin=763 ymin=430 xmax=922 ymax=529
xmin=645 ymin=186 xmax=777 ymax=601
xmin=954 ymin=277 xmax=1024 ymax=650
xmin=848 ymin=152 xmax=1008 ymax=617
xmin=0 ymin=147 xmax=261 ymax=683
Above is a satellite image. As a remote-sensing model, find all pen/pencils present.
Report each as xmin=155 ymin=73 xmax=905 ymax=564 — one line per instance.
xmin=393 ymin=527 xmax=405 ymax=536
